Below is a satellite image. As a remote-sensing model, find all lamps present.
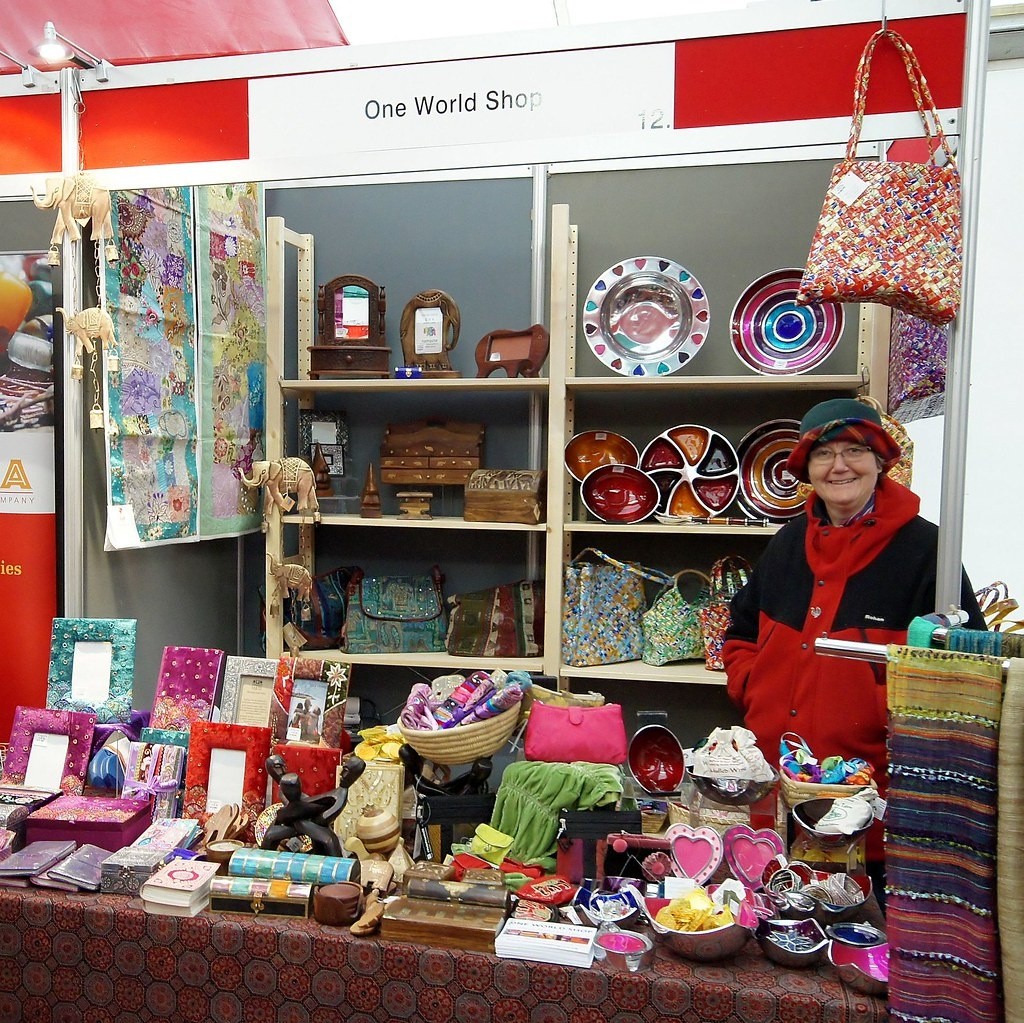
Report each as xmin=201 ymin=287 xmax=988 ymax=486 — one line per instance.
xmin=27 ymin=20 xmax=101 ymax=63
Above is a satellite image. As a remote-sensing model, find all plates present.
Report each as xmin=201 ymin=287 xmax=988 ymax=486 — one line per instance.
xmin=735 ymin=418 xmax=813 ymax=524
xmin=637 ymin=425 xmax=740 ymax=518
xmin=581 ymin=257 xmax=710 ymax=377
xmin=729 ymin=271 xmax=846 ymax=376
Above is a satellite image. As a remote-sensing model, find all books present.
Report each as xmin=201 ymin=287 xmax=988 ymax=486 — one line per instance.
xmin=138 ymin=859 xmax=222 ymax=917
xmin=495 ymin=918 xmax=597 ymax=968
xmin=130 ymin=818 xmax=205 ymax=850
xmin=0 ymin=841 xmax=114 ymax=891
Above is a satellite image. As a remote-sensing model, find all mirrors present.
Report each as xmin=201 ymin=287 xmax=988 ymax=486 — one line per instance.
xmin=319 ymin=275 xmax=386 ymax=346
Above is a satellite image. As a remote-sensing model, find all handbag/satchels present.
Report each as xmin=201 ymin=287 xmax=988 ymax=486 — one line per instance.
xmin=795 ymin=29 xmax=961 ymax=327
xmin=285 ymin=543 xmax=756 ymax=672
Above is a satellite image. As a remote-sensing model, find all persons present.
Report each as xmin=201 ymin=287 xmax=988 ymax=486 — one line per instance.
xmin=260 ymin=754 xmax=366 ymax=858
xmin=723 ymin=399 xmax=989 ymax=920
xmin=399 ymin=744 xmax=493 ymax=797
xmin=288 ymin=700 xmax=321 ymax=736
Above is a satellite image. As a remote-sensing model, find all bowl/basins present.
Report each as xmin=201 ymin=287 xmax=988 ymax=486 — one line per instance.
xmin=580 ymin=464 xmax=660 ymax=524
xmin=594 ymin=932 xmax=652 ymax=971
xmin=827 ymin=937 xmax=891 ymax=994
xmin=686 ymin=763 xmax=778 ymax=803
xmin=206 ymin=839 xmax=245 ymax=876
xmin=581 ymin=904 xmax=639 ymax=929
xmin=630 ymin=725 xmax=683 ymax=794
xmin=755 ymin=918 xmax=829 ymax=966
xmin=313 ymin=880 xmax=361 ymax=925
xmin=565 ymin=430 xmax=639 ymax=482
xmin=644 ymin=898 xmax=752 ymax=957
xmin=761 ymin=862 xmax=871 ymax=923
xmin=793 ymin=796 xmax=874 ymax=845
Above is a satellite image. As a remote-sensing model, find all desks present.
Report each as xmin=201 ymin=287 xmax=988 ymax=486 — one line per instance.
xmin=1 ymin=885 xmax=892 ymax=1022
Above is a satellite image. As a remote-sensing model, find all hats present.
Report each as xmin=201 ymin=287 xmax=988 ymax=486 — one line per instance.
xmin=785 ymin=399 xmax=902 ymax=486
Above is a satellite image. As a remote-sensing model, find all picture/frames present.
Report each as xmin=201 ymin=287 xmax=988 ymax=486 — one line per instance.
xmin=219 ymin=655 xmax=279 ymax=728
xmin=1 ymin=705 xmax=98 ymax=797
xmin=300 ymin=409 xmax=351 ymax=477
xmin=47 ymin=617 xmax=138 ymax=724
xmin=268 ymin=657 xmax=351 ymax=747
xmin=182 ymin=721 xmax=272 ymax=844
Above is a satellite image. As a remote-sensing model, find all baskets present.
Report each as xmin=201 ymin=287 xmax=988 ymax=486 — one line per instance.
xmin=395 ymin=687 xmax=521 ymax=763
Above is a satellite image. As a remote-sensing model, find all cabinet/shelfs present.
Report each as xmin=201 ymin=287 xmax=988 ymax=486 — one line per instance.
xmin=307 ymin=346 xmax=392 ymax=380
xmin=381 ymin=414 xmax=485 ymax=485
xmin=263 ymin=202 xmax=891 ymax=697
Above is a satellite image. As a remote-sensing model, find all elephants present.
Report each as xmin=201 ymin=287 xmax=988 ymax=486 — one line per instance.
xmin=56 ymin=307 xmax=119 ymax=356
xmin=236 ymin=457 xmax=321 ymax=513
xmin=266 ymin=550 xmax=314 ymax=603
xmin=29 ymin=172 xmax=115 ymax=246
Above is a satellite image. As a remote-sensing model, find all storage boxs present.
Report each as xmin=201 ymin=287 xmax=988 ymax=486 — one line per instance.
xmin=463 ymin=468 xmax=547 ymax=525
xmin=0 ymin=728 xmax=188 ymax=852
xmin=403 ymin=861 xmax=506 ymax=894
xmin=209 ymin=874 xmax=314 ymax=920
xmin=100 ymin=846 xmax=173 ymax=896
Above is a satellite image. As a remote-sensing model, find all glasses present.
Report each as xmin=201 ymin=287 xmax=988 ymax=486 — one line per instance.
xmin=810 ymin=445 xmax=872 ymax=459
